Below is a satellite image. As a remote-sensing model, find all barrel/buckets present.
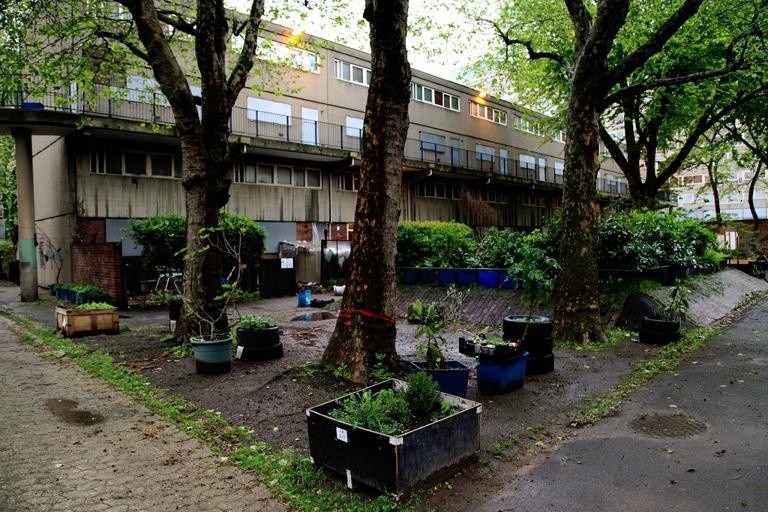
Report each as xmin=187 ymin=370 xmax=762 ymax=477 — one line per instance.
xmin=189 ymin=332 xmax=231 ymax=376
xmin=298 ymin=290 xmax=311 ymax=307
xmin=503 ymin=312 xmax=554 ymax=373
xmin=169 ymin=300 xmax=182 ymax=330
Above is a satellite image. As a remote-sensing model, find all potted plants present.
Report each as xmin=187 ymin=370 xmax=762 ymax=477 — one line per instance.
xmin=171 ymin=215 xmax=247 ymax=375
xmin=54 ymin=301 xmax=120 ymax=338
xmin=48 ymin=282 xmax=106 ymax=304
xmin=305 ymin=376 xmax=482 ymax=493
xmin=236 ymin=314 xmax=285 ymax=360
xmin=637 ymin=277 xmax=692 ymax=346
xmin=471 ymin=218 xmax=559 ymax=389
xmin=400 ymin=299 xmax=472 ymax=396
